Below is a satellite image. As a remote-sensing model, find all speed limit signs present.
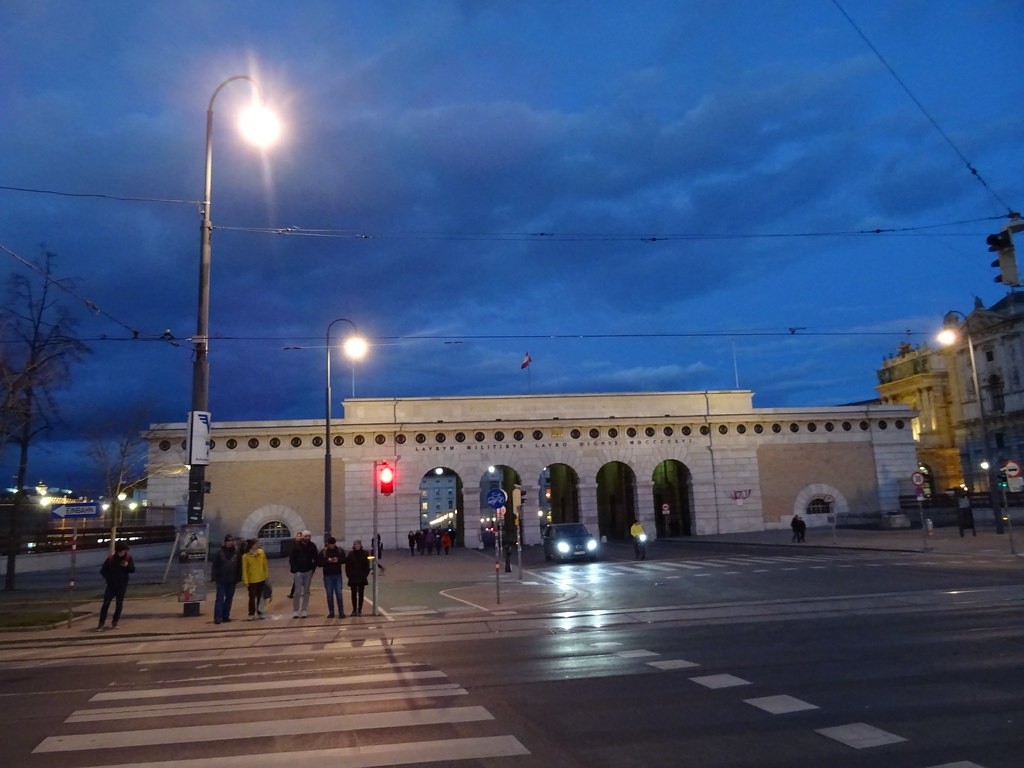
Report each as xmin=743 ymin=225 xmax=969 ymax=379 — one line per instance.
xmin=911 ymin=472 xmax=924 ymax=485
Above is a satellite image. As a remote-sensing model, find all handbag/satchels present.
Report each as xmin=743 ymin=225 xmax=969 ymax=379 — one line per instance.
xmin=263 ymin=583 xmax=273 ymax=599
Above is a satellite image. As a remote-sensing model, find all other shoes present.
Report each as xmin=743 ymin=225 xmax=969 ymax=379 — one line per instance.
xmin=215 ymin=618 xmax=221 ymax=624
xmin=301 ymin=610 xmax=308 ymax=617
xmin=222 ymin=618 xmax=233 ymax=622
xmin=247 ymin=616 xmax=256 ymax=622
xmin=259 ymin=615 xmax=266 ymax=619
xmin=351 ymin=611 xmax=356 ymax=617
xmin=327 ymin=613 xmax=334 ymax=618
xmin=112 ymin=625 xmax=120 ymax=630
xmin=292 ymin=611 xmax=299 ymax=618
xmin=95 ymin=626 xmax=105 ymax=632
xmin=357 ymin=611 xmax=363 ymax=618
xmin=339 ymin=613 xmax=345 ymax=618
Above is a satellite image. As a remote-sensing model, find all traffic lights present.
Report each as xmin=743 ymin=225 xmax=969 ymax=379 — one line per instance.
xmin=987 ymin=229 xmax=1017 ymax=286
xmin=381 ymin=467 xmax=393 ymax=494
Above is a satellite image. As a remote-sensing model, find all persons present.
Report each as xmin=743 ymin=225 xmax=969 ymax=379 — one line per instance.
xmin=371 ymin=534 xmax=385 ymax=575
xmin=631 ymin=520 xmax=645 ymax=538
xmin=408 ymin=529 xmax=456 ymax=556
xmin=211 ymin=534 xmax=242 ymax=623
xmin=791 ymin=515 xmax=806 ymax=543
xmin=289 ymin=531 xmax=319 ymax=618
xmin=345 ymin=540 xmax=370 ymax=616
xmin=96 ymin=543 xmax=135 ymax=632
xmin=241 ymin=539 xmax=269 ymax=619
xmin=317 ymin=537 xmax=347 ymax=618
xmin=287 ymin=532 xmax=302 ymax=598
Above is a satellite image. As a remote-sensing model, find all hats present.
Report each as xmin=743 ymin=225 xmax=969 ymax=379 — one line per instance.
xmin=354 ymin=541 xmax=361 ymax=545
xmin=225 ymin=535 xmax=234 ymax=542
xmin=301 ymin=530 xmax=312 ymax=540
xmin=326 ymin=537 xmax=336 ymax=545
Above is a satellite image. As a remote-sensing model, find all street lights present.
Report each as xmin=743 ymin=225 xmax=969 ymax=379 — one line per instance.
xmin=182 ymin=74 xmax=284 ymax=615
xmin=937 ymin=310 xmax=1004 ymax=533
xmin=324 ymin=318 xmax=367 ymax=540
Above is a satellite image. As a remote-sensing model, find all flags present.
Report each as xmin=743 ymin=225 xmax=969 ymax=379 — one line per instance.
xmin=521 ymin=354 xmax=531 ymax=369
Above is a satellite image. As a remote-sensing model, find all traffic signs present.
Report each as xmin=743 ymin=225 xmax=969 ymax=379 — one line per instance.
xmin=50 ymin=502 xmax=100 ymax=519
